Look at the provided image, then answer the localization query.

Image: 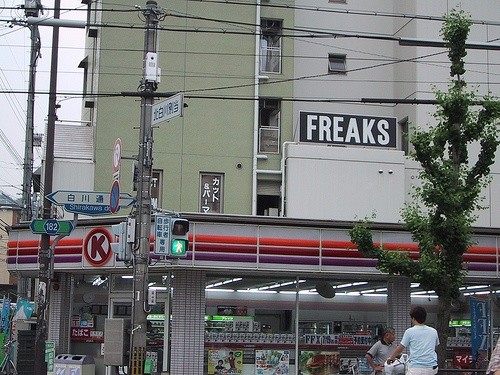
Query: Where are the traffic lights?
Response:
[169,218,190,258]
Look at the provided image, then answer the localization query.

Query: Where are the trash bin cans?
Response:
[54,354,95,375]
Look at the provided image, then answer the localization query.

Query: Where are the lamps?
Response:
[410,282,500,299]
[206,278,387,295]
[92,276,108,289]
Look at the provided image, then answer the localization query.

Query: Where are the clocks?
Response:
[82,293,95,303]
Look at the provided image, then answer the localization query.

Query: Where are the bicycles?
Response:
[0,340,19,375]
[386,356,411,375]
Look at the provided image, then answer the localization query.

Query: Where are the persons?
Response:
[388,306,441,375]
[365,327,402,375]
[225,352,237,368]
[485,334,500,375]
[215,360,225,372]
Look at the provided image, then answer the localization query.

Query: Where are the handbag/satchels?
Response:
[384,354,409,375]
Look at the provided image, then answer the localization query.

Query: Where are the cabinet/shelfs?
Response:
[146,315,225,339]
[447,327,472,370]
